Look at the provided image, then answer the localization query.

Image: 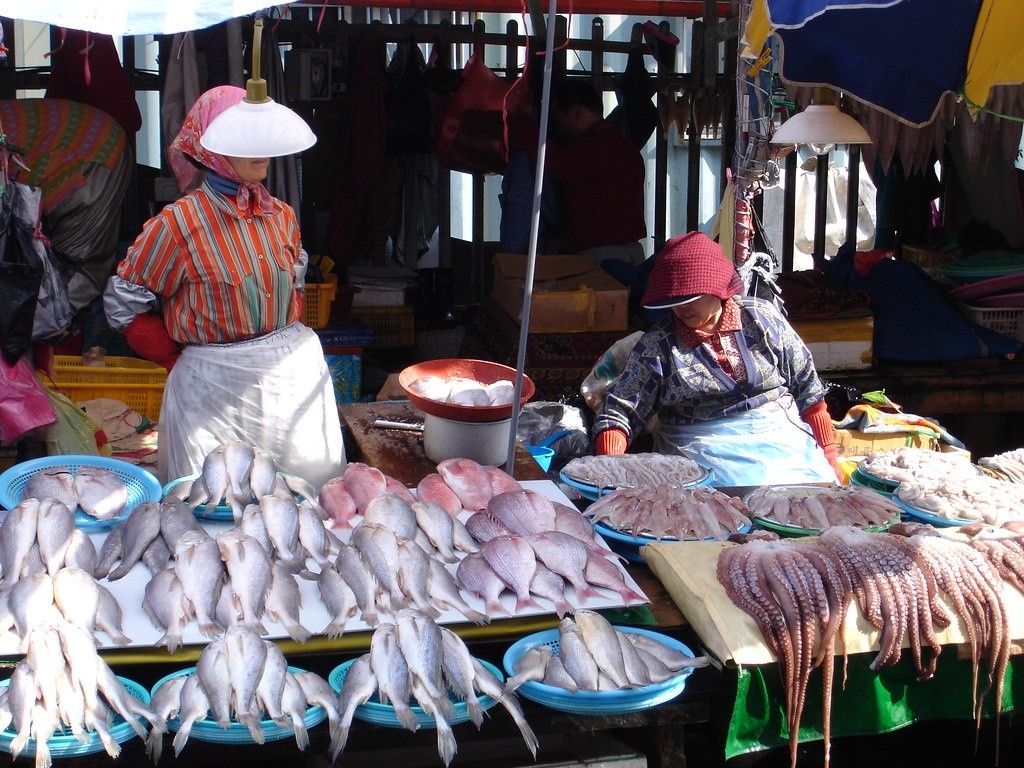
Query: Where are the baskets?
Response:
[303,275,339,330]
[957,303,1024,338]
[40,371,169,421]
[47,355,167,372]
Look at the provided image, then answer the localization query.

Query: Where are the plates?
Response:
[559,461,715,502]
[740,484,900,539]
[163,472,313,520]
[584,509,752,563]
[850,460,901,499]
[891,485,977,527]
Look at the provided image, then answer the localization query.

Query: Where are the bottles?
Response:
[769,73,789,130]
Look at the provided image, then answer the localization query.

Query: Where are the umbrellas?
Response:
[739,0,1024,130]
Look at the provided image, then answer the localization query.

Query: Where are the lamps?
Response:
[769,87,873,157]
[200,17,317,160]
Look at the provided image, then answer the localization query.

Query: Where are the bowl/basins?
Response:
[414,406,523,467]
[398,358,535,422]
[524,445,554,474]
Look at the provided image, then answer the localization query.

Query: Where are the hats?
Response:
[555,83,606,116]
[642,294,703,310]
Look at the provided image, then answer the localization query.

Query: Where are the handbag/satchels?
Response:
[33,238,73,343]
[0,183,45,367]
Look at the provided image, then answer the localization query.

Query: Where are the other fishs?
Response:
[750,484,905,526]
[1,372,750,766]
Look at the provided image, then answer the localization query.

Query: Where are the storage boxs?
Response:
[351,284,404,306]
[494,254,629,331]
[300,274,336,329]
[787,315,875,371]
[35,354,168,424]
[360,307,416,348]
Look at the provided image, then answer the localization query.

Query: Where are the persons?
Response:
[0,98,127,356]
[592,231,845,489]
[102,84,346,495]
[509,84,647,268]
